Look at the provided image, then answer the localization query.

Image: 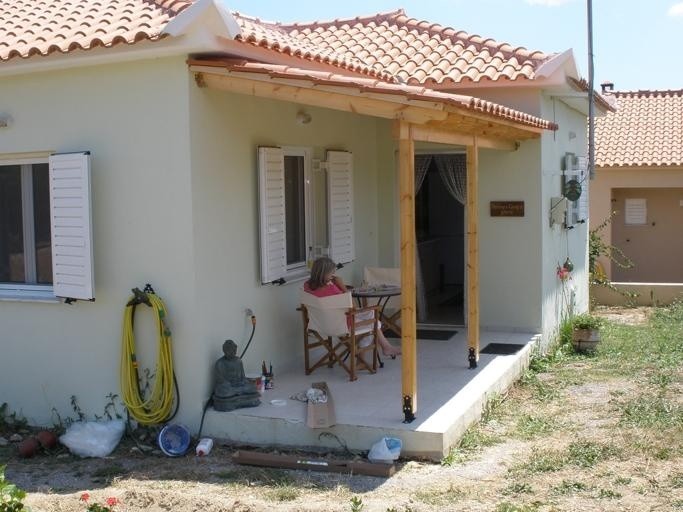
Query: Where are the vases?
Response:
[16,429,56,458]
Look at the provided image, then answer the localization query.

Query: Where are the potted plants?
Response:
[571,311,606,352]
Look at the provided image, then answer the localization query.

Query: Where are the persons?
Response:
[214,339,258,398]
[303,257,401,370]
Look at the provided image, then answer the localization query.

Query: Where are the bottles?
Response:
[196,439,212,458]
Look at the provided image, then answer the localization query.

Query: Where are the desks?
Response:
[339,285,401,369]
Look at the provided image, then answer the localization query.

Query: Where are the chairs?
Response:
[296,303,384,382]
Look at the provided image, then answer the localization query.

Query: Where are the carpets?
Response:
[381,328,458,341]
[479,343,525,356]
